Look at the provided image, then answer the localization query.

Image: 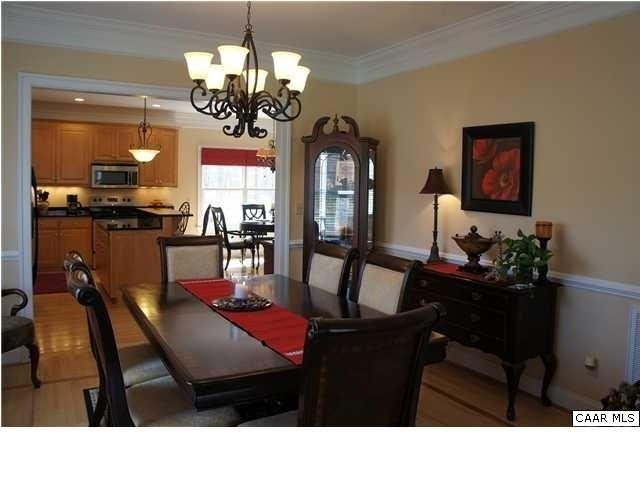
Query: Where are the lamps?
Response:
[128,97,161,164]
[256,120,275,173]
[183,1,311,138]
[533,221,552,285]
[419,168,453,263]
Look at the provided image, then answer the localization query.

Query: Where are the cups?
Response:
[66,194,84,214]
[231,274,249,300]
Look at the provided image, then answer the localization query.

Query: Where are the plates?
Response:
[212,295,273,313]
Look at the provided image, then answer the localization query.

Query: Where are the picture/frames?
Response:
[461,122,534,216]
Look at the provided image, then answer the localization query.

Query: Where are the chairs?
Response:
[2,202,445,426]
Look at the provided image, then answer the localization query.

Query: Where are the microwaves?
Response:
[91,163,139,188]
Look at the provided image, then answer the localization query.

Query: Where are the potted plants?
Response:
[502,229,552,284]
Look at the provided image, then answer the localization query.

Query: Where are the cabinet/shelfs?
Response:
[139,126,179,187]
[421,262,561,420]
[32,121,93,186]
[93,123,139,162]
[301,113,379,280]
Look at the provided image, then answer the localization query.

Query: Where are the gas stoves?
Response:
[88,207,154,219]
